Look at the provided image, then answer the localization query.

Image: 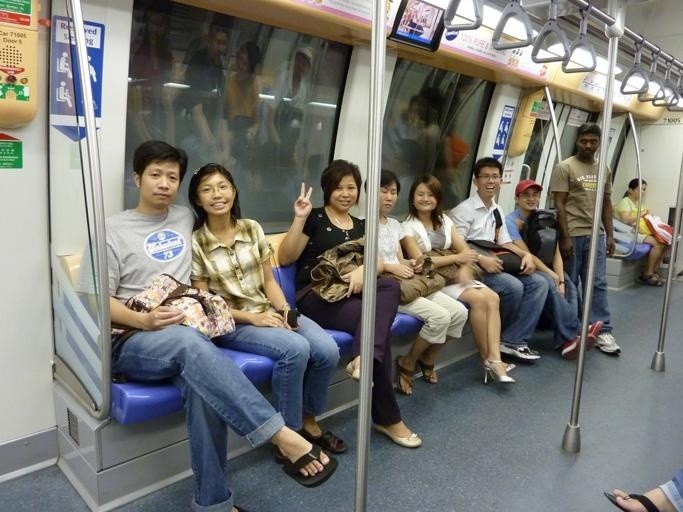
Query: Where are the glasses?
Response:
[198,184,232,195]
[477,174,501,180]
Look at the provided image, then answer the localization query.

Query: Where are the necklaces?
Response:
[331,210,353,240]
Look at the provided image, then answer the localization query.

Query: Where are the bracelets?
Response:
[559,281,566,284]
[477,255,481,261]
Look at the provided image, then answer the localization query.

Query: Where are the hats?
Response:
[513,179,544,196]
[577,120,602,137]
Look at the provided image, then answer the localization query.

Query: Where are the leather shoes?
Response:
[344,357,375,388]
[370,419,423,450]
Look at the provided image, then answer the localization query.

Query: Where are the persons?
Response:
[188,161,348,464]
[364,170,469,395]
[277,159,423,447]
[551,125,622,354]
[403,174,516,385]
[506,179,604,359]
[403,86,471,203]
[76,140,339,512]
[613,179,666,286]
[448,158,550,360]
[133,17,325,199]
[604,470,683,511]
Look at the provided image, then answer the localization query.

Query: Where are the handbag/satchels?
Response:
[464,236,526,276]
[307,234,366,306]
[421,247,483,287]
[643,213,681,247]
[377,253,447,306]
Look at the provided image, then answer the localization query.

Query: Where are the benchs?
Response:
[274,265,300,309]
[610,211,651,258]
[326,330,355,358]
[390,312,420,337]
[110,358,183,428]
[221,349,277,385]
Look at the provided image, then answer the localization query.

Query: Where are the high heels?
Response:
[481,360,516,386]
[503,362,516,374]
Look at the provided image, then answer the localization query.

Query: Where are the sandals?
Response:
[271,446,291,465]
[652,271,666,283]
[634,275,663,287]
[297,427,349,456]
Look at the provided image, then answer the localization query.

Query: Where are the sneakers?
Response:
[498,336,541,361]
[560,334,596,360]
[586,320,604,338]
[592,332,622,355]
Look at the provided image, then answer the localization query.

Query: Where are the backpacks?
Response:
[123,272,236,346]
[521,208,561,267]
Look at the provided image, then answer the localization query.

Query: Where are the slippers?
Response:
[267,431,341,489]
[394,354,413,398]
[603,490,659,512]
[417,359,439,384]
